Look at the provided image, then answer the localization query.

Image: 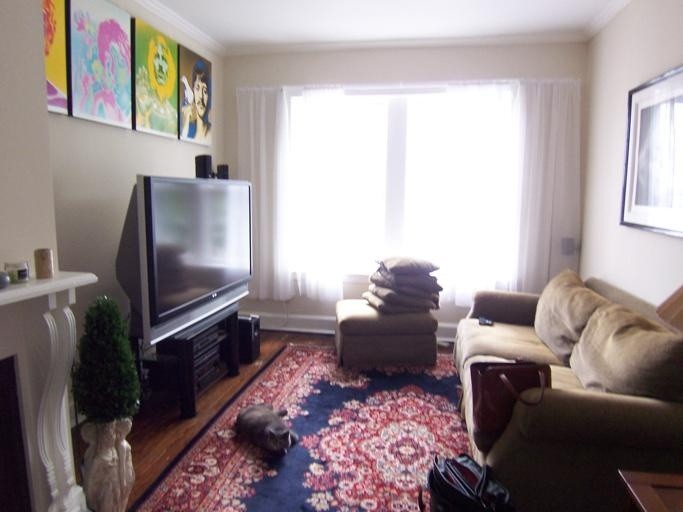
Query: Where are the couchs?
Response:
[453,278,683,512]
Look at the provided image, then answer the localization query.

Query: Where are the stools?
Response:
[335,298,439,370]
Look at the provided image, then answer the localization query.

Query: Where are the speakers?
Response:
[217,164,229,179]
[195,155,214,178]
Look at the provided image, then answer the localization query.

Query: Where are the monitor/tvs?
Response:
[137,174,253,351]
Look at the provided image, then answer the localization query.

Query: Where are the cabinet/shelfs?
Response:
[173,303,240,418]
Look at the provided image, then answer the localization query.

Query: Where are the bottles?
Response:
[4,260,30,284]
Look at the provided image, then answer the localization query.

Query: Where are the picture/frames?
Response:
[71,0,134,130]
[42,1,71,116]
[135,17,180,138]
[180,44,212,148]
[619,65,682,237]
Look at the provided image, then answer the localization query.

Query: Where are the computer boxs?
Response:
[237,314,261,363]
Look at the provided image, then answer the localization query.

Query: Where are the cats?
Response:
[236,404,299,455]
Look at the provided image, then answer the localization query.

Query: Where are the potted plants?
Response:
[71,295,141,512]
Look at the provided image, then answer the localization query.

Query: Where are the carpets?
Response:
[124,342,474,512]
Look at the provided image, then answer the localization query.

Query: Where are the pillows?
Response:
[370,271,440,300]
[377,265,443,292]
[534,270,609,364]
[375,256,440,274]
[568,306,683,399]
[369,284,437,310]
[362,292,430,314]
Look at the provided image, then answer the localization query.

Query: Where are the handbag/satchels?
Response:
[470,358,552,456]
[427,452,510,512]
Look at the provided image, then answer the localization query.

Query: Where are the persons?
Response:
[140,35,178,132]
[180,59,212,140]
